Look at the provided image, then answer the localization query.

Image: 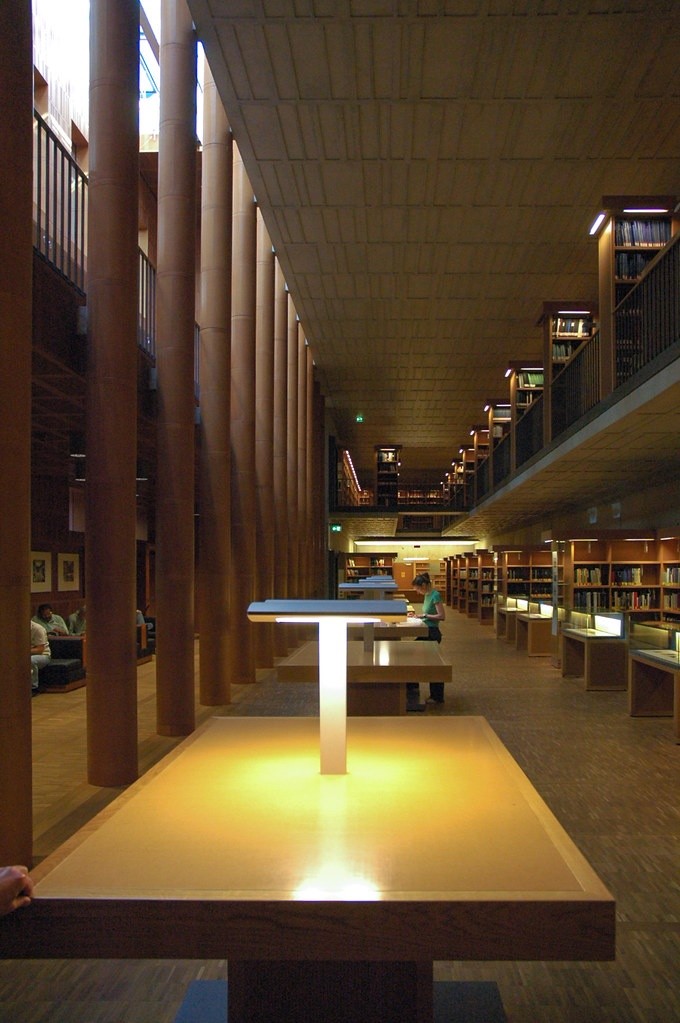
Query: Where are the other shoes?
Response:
[31,689,38,696]
[425,697,437,704]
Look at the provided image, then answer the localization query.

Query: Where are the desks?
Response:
[516,613,560,657]
[562,629,628,692]
[276,605,453,715]
[0,715,615,1023]
[497,607,527,644]
[628,649,680,746]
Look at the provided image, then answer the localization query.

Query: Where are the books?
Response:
[345,558,680,624]
[337,217,673,510]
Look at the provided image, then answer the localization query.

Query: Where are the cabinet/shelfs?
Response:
[446,526,680,630]
[342,553,398,582]
[373,445,399,505]
[413,561,446,590]
[443,195,679,505]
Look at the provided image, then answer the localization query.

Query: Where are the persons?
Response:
[407,572,446,704]
[30,605,151,697]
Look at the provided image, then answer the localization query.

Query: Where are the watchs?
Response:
[423,614,427,618]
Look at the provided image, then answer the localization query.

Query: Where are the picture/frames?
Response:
[57,552,80,592]
[30,551,52,593]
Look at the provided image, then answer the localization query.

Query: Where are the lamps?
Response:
[340,576,398,651]
[247,600,407,774]
[75,462,86,482]
[69,432,86,457]
[136,461,150,481]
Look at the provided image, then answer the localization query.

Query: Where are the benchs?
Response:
[137,616,156,665]
[38,636,86,693]
